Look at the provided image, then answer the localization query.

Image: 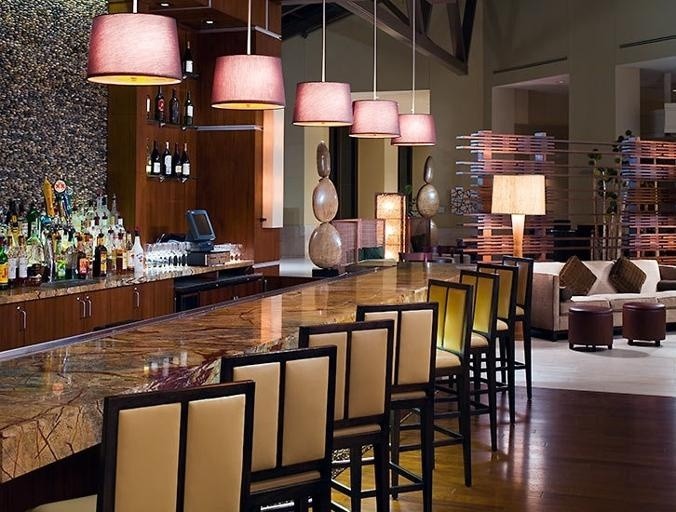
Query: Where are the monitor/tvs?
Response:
[185,210,215,239]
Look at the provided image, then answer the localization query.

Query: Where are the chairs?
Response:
[356,295,439,512]
[470,251,537,408]
[415,261,499,456]
[300,319,393,512]
[389,271,476,489]
[39,378,256,512]
[215,348,336,512]
[467,257,521,425]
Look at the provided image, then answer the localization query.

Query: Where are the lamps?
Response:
[83,1,182,87]
[256,102,284,231]
[344,0,399,141]
[211,0,288,110]
[291,0,354,125]
[374,192,409,262]
[388,0,436,147]
[483,172,549,261]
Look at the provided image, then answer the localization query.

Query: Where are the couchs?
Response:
[526,259,676,341]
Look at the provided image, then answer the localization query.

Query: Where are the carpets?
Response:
[456,332,676,401]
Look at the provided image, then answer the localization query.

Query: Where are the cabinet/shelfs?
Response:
[1,303,27,350]
[145,116,199,183]
[197,276,260,305]
[26,288,109,348]
[110,280,176,324]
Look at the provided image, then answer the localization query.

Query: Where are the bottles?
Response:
[150,140,160,175]
[214,243,240,262]
[169,86,181,124]
[154,85,164,121]
[0,194,142,289]
[145,242,186,268]
[172,143,182,178]
[184,89,193,126]
[182,40,193,75]
[161,141,172,177]
[180,143,191,178]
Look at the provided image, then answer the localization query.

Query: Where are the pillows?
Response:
[612,253,647,293]
[558,255,598,296]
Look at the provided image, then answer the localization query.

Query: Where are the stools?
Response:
[564,302,616,353]
[621,300,668,347]
